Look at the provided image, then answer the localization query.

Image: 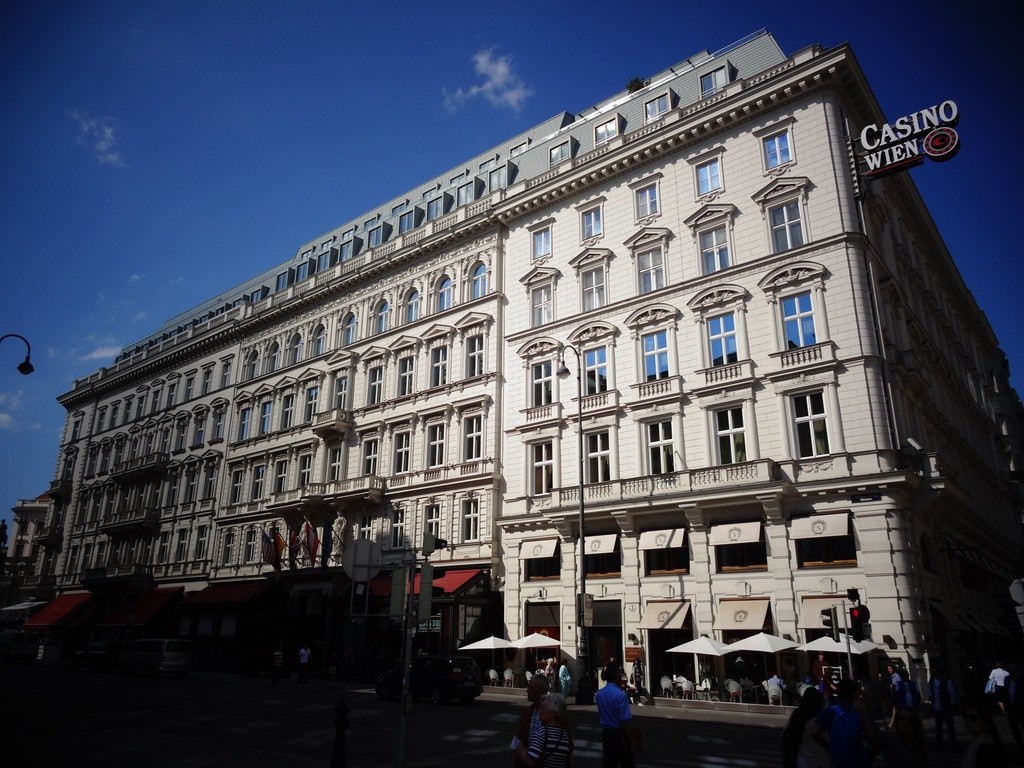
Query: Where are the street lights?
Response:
[557,344,596,706]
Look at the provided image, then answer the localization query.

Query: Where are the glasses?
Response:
[962,713,980,722]
[895,714,915,722]
[527,684,536,690]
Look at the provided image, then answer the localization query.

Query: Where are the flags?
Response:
[262,521,332,581]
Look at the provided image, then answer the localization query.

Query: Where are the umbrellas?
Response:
[664,632,880,688]
[457,631,561,670]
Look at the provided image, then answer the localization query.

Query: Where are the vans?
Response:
[117,637,194,677]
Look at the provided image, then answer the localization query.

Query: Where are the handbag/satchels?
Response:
[624,727,643,752]
[984,678,995,694]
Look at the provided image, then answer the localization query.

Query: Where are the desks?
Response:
[672,680,761,703]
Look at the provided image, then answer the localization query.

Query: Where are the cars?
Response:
[376,652,483,705]
[75,642,117,669]
[10,628,41,657]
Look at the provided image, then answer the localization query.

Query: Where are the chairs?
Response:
[695,678,712,701]
[503,670,514,688]
[489,669,498,686]
[525,671,533,682]
[681,681,694,699]
[768,685,783,705]
[660,677,673,697]
[675,676,687,698]
[727,681,743,702]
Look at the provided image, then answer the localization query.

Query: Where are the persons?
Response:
[595,662,639,768]
[297,643,313,684]
[0,519,8,548]
[761,651,1024,768]
[509,673,574,768]
[326,505,348,568]
[297,511,317,569]
[544,658,754,703]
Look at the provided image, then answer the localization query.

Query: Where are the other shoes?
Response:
[931,742,943,749]
[949,739,956,746]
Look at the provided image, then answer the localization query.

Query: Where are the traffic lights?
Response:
[423,530,447,557]
[820,606,839,642]
[420,562,448,626]
[848,606,870,643]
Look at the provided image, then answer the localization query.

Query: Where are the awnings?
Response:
[95,587,183,631]
[797,597,859,629]
[711,600,772,629]
[636,602,690,629]
[790,513,854,539]
[638,528,688,549]
[0,601,50,611]
[518,539,560,559]
[178,582,277,608]
[575,535,620,553]
[709,522,765,546]
[22,593,96,632]
[369,569,485,608]
